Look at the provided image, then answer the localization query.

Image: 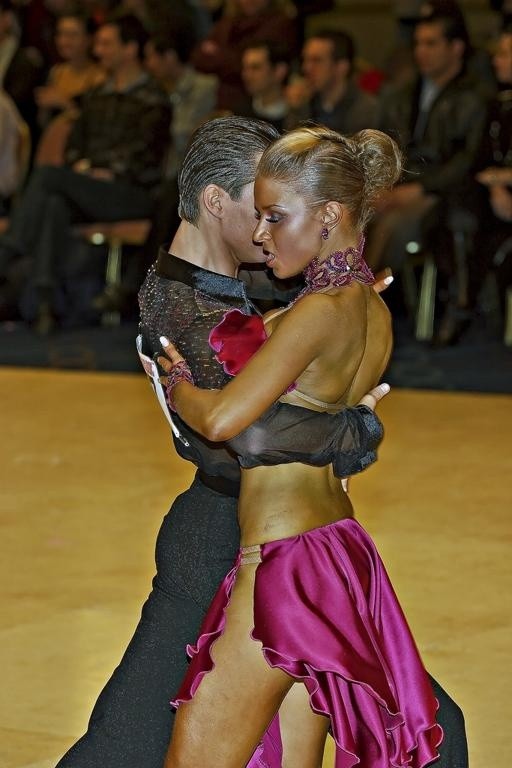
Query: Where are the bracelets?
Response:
[161,360,197,414]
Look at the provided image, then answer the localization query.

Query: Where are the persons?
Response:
[0,2,512,355]
[132,126,446,767]
[56,111,470,764]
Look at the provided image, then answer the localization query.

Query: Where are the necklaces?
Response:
[283,237,376,306]
